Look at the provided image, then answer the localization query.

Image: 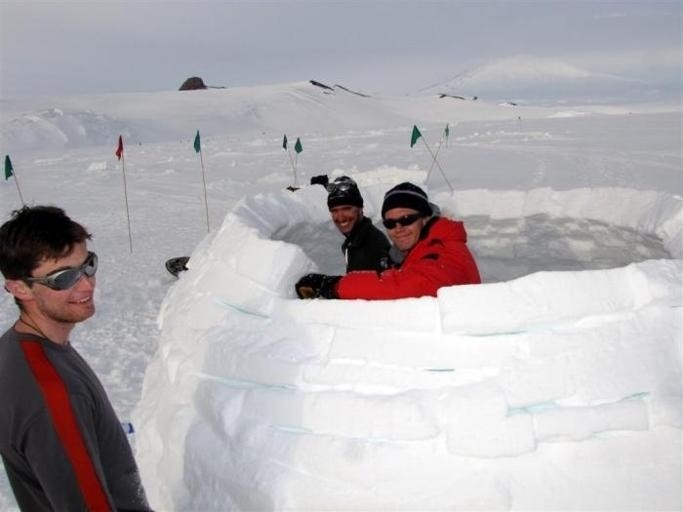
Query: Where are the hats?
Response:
[382,183,432,219]
[328,176,363,208]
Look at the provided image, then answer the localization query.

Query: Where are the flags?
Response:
[4,155,13,182]
[410,125,421,148]
[193,130,201,153]
[115,135,125,161]
[282,134,288,149]
[294,137,303,154]
[444,124,450,147]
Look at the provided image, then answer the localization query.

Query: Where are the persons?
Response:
[325,175,392,272]
[293,181,482,301]
[1,204,154,511]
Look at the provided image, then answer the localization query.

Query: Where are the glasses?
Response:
[324,183,357,192]
[21,251,98,290]
[383,213,427,229]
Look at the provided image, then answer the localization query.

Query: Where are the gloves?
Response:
[295,274,343,298]
